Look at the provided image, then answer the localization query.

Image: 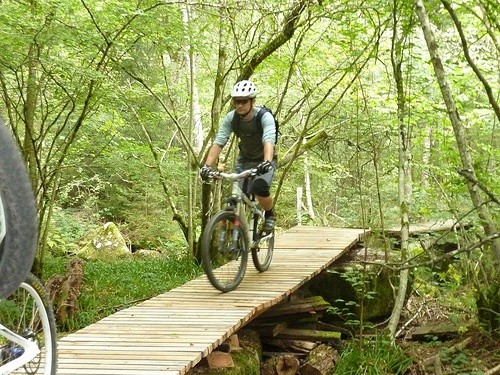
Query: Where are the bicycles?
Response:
[0,271,58,375]
[200,168,275,292]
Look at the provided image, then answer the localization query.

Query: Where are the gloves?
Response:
[257,160,273,176]
[200,164,211,181]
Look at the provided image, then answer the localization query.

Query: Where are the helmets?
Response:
[232,80,257,99]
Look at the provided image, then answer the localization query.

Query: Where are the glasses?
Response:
[233,99,251,105]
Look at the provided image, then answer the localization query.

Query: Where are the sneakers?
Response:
[264,213,276,231]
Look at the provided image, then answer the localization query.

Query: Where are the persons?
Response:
[200,80,285,233]
